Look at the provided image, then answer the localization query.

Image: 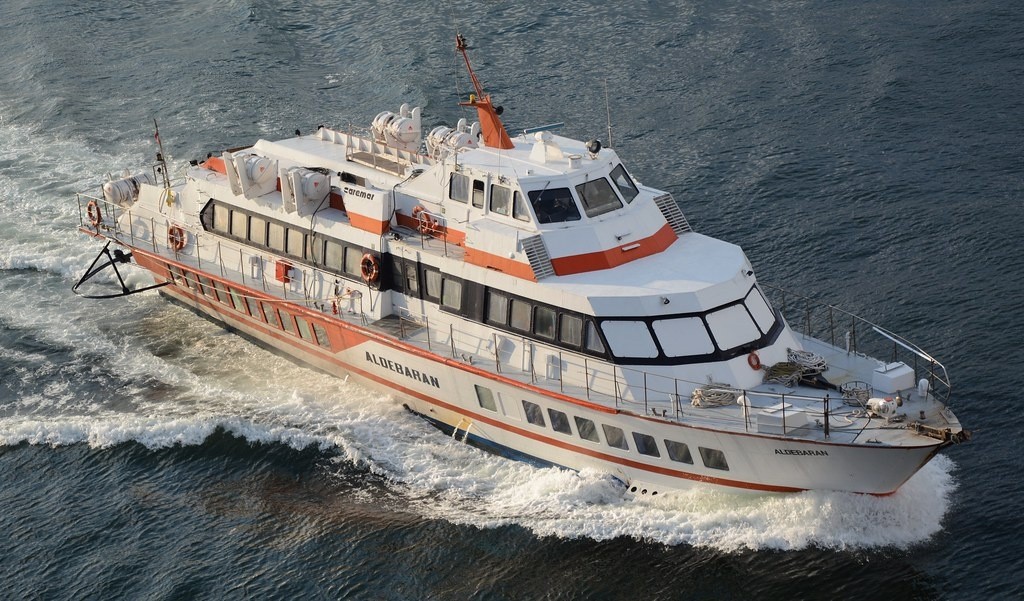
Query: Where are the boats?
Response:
[72,27,964,500]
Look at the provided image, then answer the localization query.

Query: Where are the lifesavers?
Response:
[87,201,102,228]
[169,224,183,250]
[747,354,761,370]
[411,206,432,235]
[358,253,380,282]
